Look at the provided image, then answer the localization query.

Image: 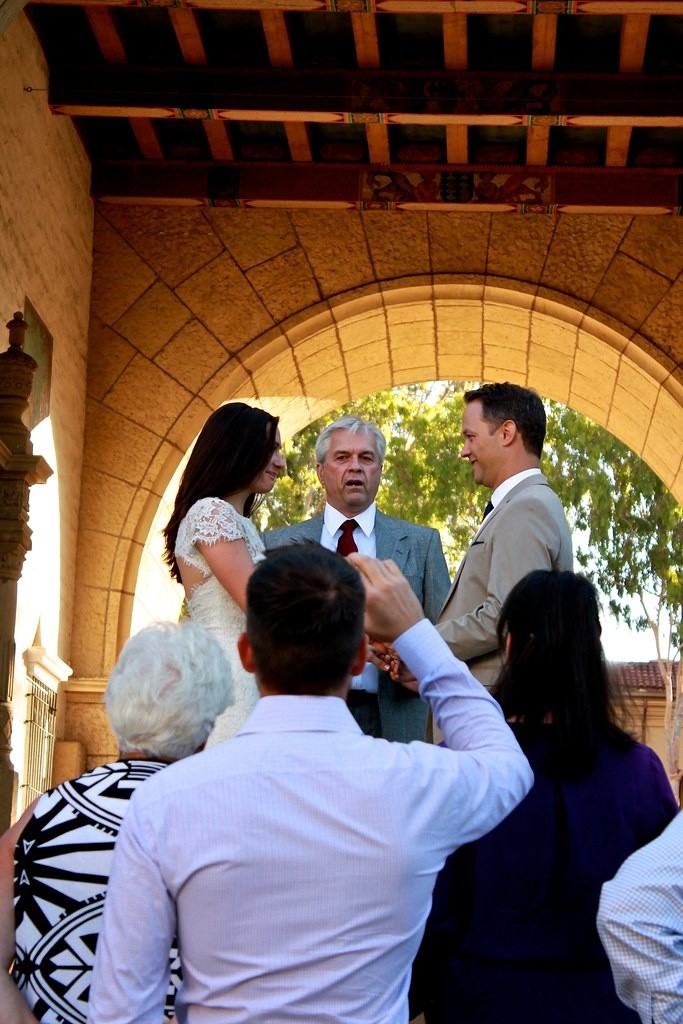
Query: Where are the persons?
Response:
[158,402,286,753]
[87,540,537,1024]
[407,568,683,1024]
[0,619,239,1024]
[254,415,452,744]
[596,808,683,1024]
[432,382,573,696]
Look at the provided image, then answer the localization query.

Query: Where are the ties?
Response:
[481,501,495,521]
[337,520,360,558]
